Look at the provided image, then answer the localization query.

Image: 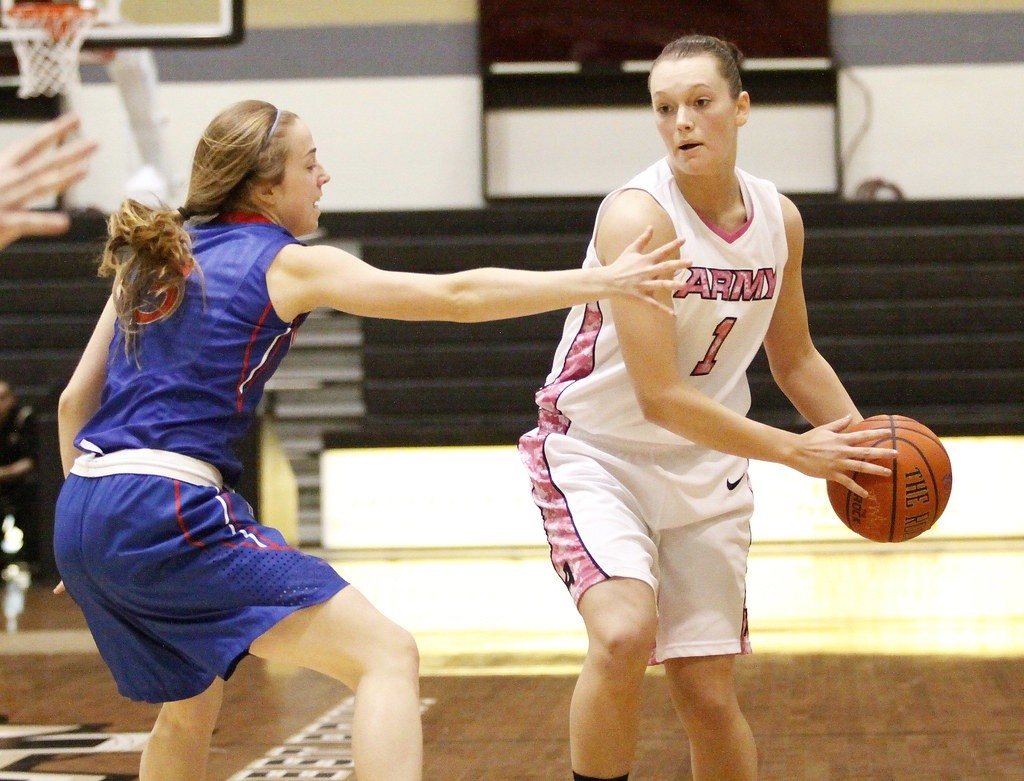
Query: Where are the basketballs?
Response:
[826,416,954,544]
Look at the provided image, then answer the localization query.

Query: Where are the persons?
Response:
[0,379,51,587]
[53,98,693,781]
[0,110,100,252]
[517,31,900,781]
